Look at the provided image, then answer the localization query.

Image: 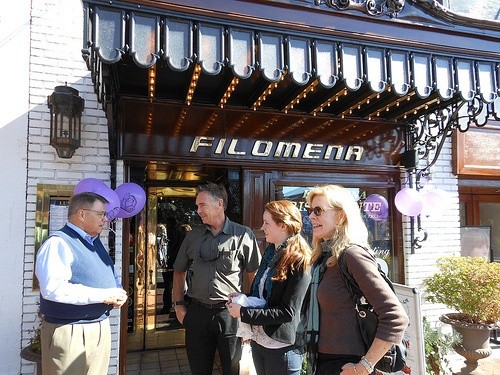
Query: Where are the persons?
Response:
[305,184,410,375]
[161,224,193,313]
[173,183,262,375]
[34,192,128,375]
[225,200,312,375]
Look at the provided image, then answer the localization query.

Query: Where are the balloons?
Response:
[394,187,423,217]
[362,193,389,221]
[75,179,147,222]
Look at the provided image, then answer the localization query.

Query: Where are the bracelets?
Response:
[172,301,188,310]
[359,356,374,374]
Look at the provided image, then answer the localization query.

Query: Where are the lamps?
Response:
[46,81,85,159]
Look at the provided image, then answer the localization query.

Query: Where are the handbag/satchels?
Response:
[337,244,411,374]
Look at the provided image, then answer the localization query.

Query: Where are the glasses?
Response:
[82,208,107,218]
[198,237,220,262]
[307,206,327,217]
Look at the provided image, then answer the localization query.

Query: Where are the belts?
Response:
[189,297,226,311]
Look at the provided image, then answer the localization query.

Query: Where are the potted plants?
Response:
[418,254,500,352]
[20,301,42,375]
